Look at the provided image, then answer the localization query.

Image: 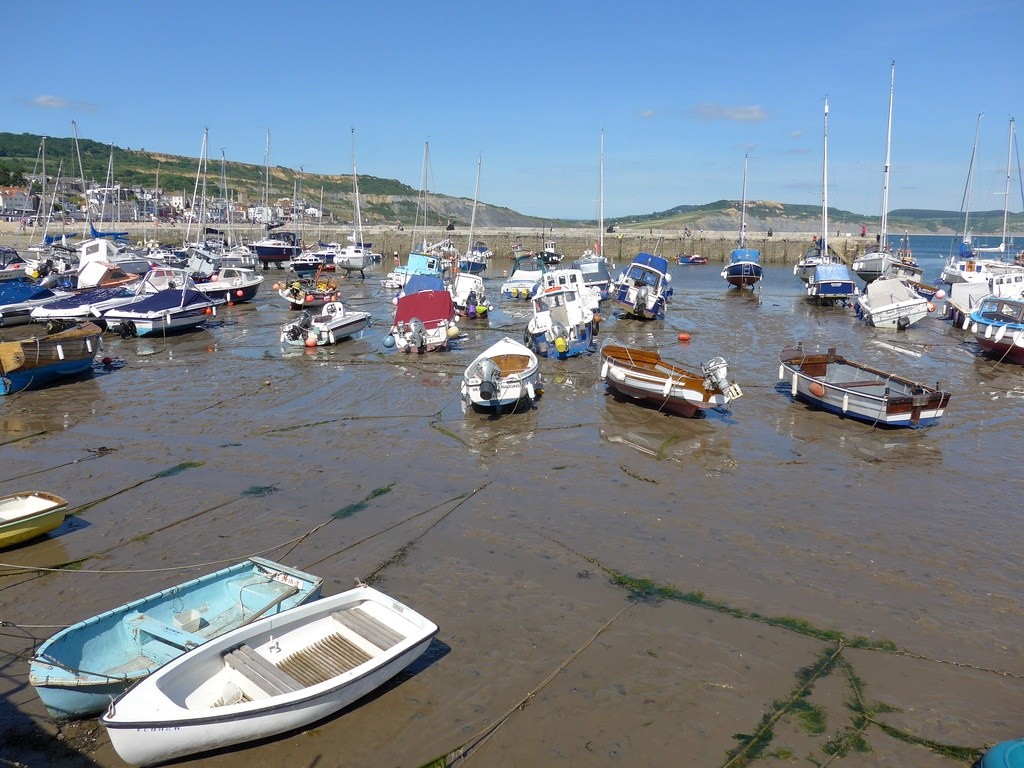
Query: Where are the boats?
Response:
[0,489,69,549]
[941,282,1024,364]
[777,341,951,430]
[853,272,937,332]
[28,554,324,723]
[499,219,616,360]
[380,252,493,354]
[460,336,546,407]
[676,254,709,265]
[599,337,744,418]
[98,576,440,767]
[805,264,858,309]
[0,238,265,397]
[606,237,674,320]
[274,274,374,347]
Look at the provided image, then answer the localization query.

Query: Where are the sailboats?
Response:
[579,128,617,269]
[12,120,188,269]
[719,154,764,286]
[405,134,493,274]
[793,98,851,281]
[939,112,1024,285]
[332,126,384,271]
[181,124,344,272]
[850,58,923,284]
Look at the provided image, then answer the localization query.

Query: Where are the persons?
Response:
[861,225,866,237]
[813,235,816,243]
[768,228,772,237]
